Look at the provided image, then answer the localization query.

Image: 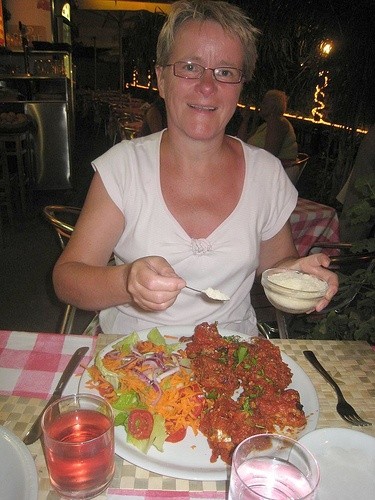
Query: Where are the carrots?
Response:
[81,340,204,437]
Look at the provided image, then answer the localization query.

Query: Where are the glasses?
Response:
[164,61,246,84]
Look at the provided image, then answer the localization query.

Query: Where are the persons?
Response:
[237,89,300,184]
[268,124,375,339]
[53,4,339,337]
[136,97,166,138]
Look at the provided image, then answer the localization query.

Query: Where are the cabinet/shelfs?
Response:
[0,50,75,190]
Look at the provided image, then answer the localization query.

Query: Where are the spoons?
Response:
[185,285,230,301]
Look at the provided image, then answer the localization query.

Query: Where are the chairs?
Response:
[294,242,375,335]
[282,152,309,183]
[60,253,289,340]
[42,206,83,250]
[0,136,19,231]
[76,89,162,147]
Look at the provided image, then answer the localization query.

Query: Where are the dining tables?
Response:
[0,330,375,500]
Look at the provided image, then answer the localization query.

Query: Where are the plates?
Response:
[77,325,319,481]
[288,428,375,500]
[0,423,38,500]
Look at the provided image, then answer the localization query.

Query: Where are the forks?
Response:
[303,351,374,426]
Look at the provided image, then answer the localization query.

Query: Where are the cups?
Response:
[40,392,117,500]
[228,433,320,500]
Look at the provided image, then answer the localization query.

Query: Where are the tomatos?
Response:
[179,384,204,400]
[165,419,186,443]
[128,410,154,440]
[186,395,208,421]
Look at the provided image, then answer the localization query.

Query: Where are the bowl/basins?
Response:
[261,268,329,314]
[0,112,33,133]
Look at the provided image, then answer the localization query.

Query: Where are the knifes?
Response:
[22,346,89,444]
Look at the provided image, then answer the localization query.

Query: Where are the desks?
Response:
[250,197,341,339]
[0,129,34,229]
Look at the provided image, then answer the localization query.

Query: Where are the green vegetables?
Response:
[95,326,274,454]
